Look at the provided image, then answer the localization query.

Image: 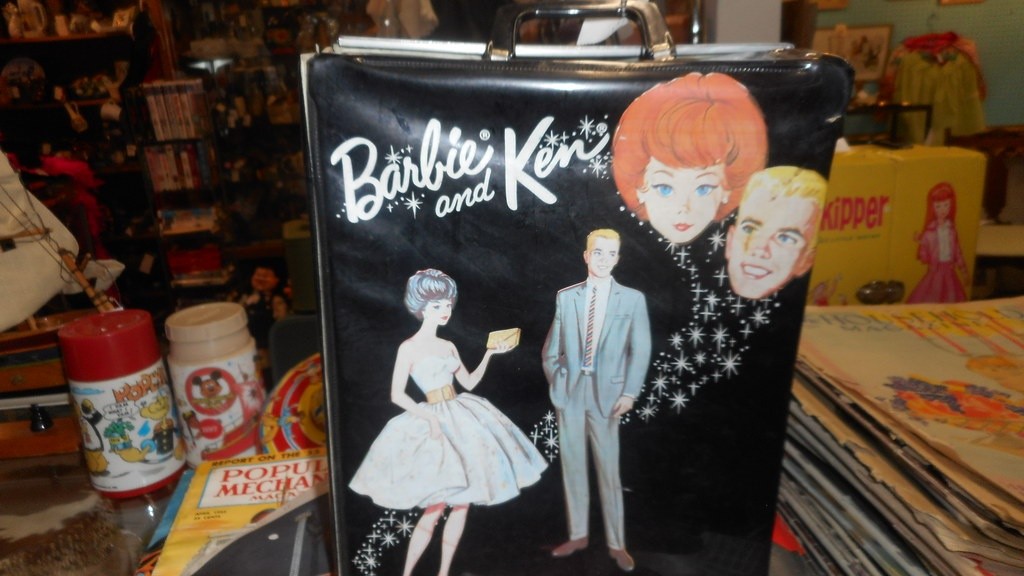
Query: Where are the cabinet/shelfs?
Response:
[0,0,336,310]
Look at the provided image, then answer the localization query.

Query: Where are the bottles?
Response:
[165,302,267,468]
[58,311,187,499]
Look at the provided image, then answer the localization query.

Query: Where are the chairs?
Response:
[267,316,323,390]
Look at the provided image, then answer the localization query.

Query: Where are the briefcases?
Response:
[300,0,854,576]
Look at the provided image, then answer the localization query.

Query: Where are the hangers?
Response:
[890,13,981,69]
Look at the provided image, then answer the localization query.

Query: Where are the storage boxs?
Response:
[803,137,987,306]
[807,23,895,85]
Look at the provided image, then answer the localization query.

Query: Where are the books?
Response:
[140,80,200,140]
[780,296,1024,576]
[144,146,198,192]
